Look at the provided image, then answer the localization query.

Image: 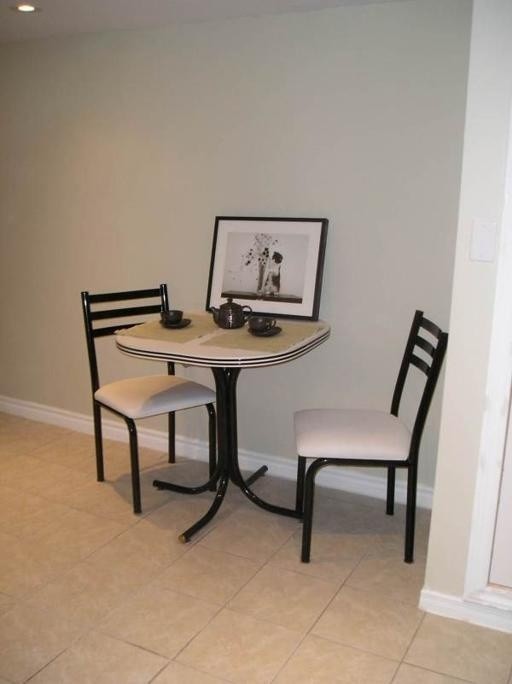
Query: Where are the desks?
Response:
[115,314,331,544]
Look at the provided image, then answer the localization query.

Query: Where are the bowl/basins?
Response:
[160,309,184,323]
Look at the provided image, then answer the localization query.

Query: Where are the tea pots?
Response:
[211,298,252,329]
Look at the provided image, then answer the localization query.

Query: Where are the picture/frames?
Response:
[206,217,329,320]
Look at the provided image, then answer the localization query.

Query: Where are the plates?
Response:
[250,326,281,336]
[159,318,191,327]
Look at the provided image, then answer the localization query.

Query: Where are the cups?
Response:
[247,316,276,329]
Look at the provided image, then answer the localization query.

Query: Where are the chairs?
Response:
[294,310,448,563]
[81,284,218,514]
